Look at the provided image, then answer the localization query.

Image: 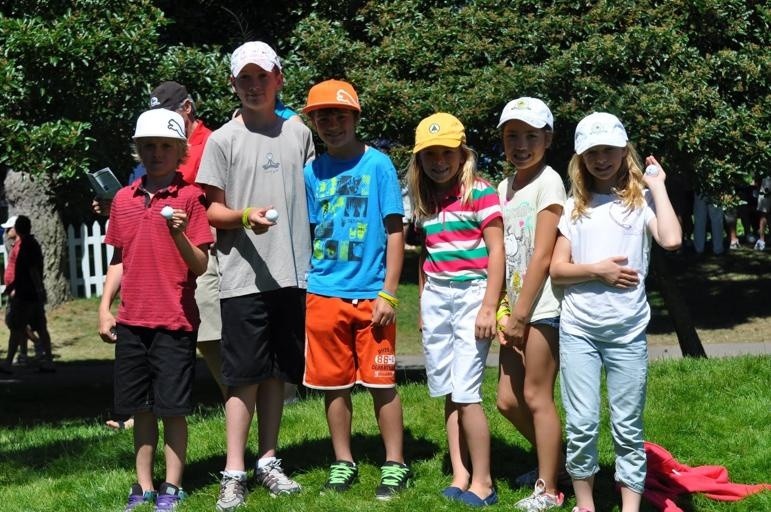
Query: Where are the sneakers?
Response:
[453,488,498,509]
[216,467,250,512]
[0,351,56,373]
[515,455,572,488]
[155,481,188,512]
[728,232,767,252]
[320,459,359,498]
[123,483,156,512]
[373,458,414,501]
[253,456,304,499]
[440,479,466,505]
[513,478,565,512]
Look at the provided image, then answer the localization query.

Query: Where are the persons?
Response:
[495,96,568,512]
[548,112,684,512]
[0,214,56,375]
[408,112,506,509]
[668,168,771,256]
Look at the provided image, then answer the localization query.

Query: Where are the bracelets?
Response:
[496,307,512,321]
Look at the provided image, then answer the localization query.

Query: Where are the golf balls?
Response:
[160,206,174,220]
[645,165,658,177]
[266,209,279,223]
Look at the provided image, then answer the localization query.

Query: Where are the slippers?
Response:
[105,416,135,432]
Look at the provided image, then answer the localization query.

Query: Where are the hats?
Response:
[573,111,628,155]
[301,79,362,118]
[412,111,467,154]
[131,107,188,142]
[150,81,188,112]
[230,40,282,79]
[0,215,19,228]
[496,96,554,136]
